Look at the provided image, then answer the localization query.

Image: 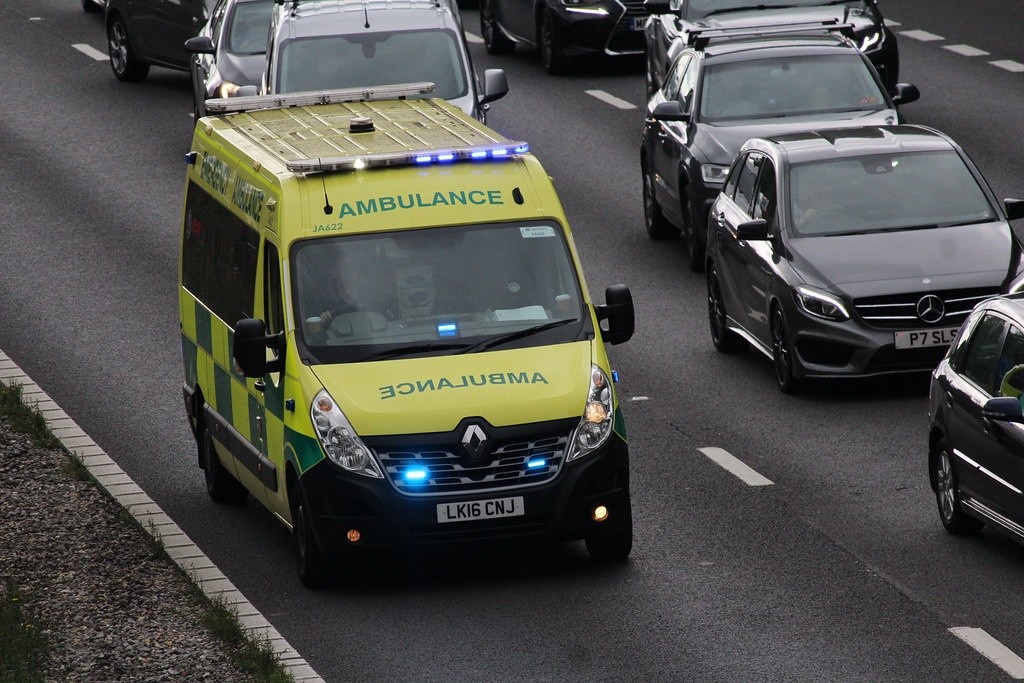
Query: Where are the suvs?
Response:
[637,23,921,275]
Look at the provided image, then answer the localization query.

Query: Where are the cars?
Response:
[642,0,900,100]
[926,293,1024,551]
[479,0,656,80]
[105,0,218,82]
[704,123,1019,399]
[183,0,278,124]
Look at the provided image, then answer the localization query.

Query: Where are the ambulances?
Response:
[179,81,634,595]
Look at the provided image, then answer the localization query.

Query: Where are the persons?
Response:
[319,256,364,327]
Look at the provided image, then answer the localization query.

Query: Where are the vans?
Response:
[236,0,511,124]
